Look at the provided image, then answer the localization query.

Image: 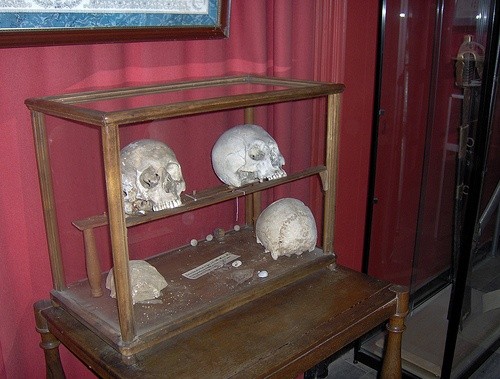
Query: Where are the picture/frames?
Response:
[0,1,231,49]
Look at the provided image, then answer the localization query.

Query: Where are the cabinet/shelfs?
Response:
[24,71,351,356]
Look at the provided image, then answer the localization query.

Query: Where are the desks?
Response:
[32,233,409,379]
